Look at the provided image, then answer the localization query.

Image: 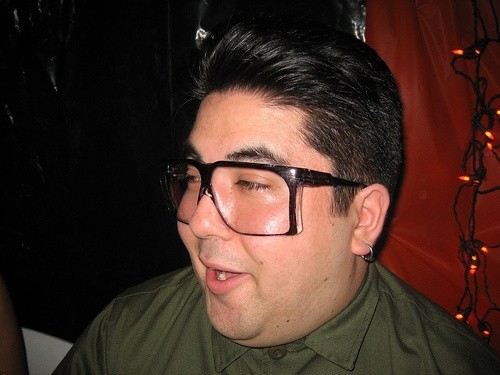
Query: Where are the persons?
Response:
[53,20,500,375]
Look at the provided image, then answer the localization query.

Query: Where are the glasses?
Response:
[158,159,368,236]
